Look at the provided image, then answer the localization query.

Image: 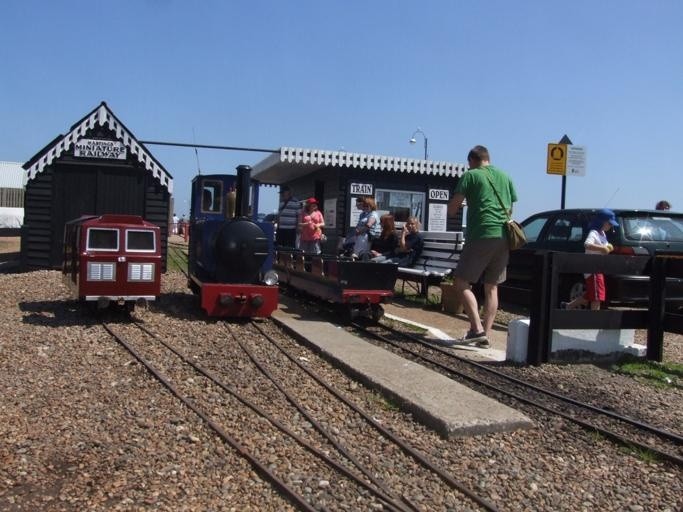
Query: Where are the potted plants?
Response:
[438,273,472,314]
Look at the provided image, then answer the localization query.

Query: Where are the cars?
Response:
[472,208,683,328]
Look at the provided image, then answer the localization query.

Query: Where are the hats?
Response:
[588,207,619,230]
[305,197,319,205]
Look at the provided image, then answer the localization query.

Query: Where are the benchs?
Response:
[379,229,464,304]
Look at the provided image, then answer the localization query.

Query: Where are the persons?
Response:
[277,187,302,247]
[656,201,670,211]
[172,214,179,233]
[343,196,422,267]
[560,209,620,311]
[297,196,325,255]
[449,146,517,349]
[180,214,187,235]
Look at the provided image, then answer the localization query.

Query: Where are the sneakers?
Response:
[559,299,570,311]
[457,328,494,348]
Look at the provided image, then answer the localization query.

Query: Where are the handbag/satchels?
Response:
[503,218,528,251]
